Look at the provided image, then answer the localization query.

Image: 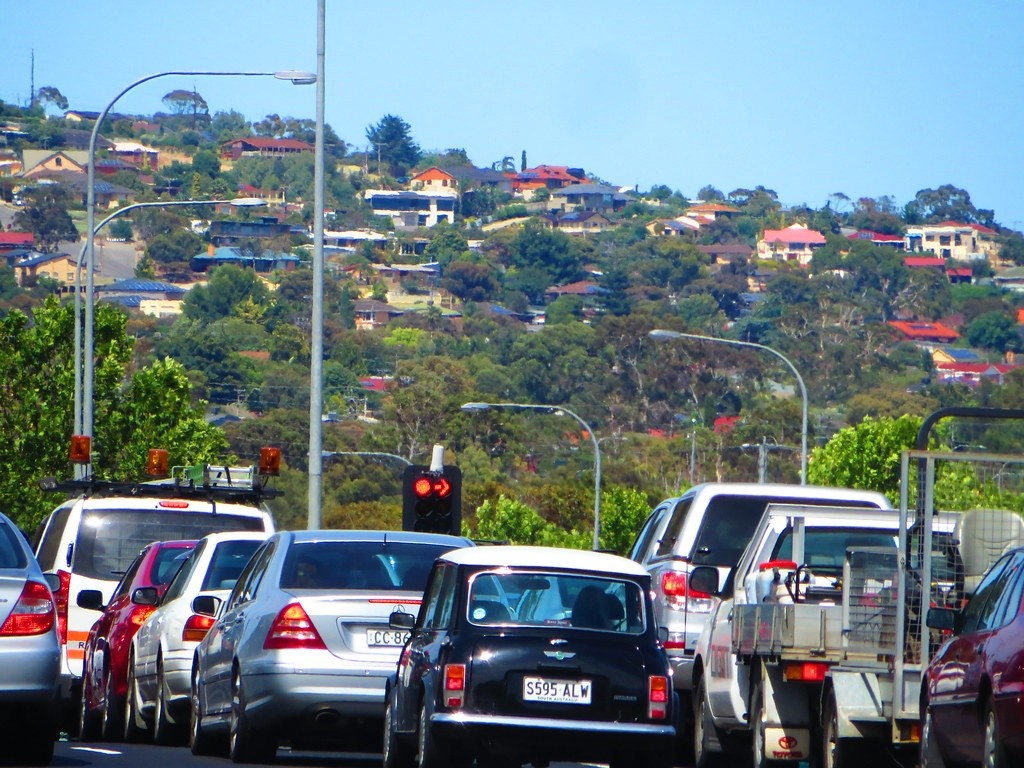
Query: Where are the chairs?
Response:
[571,592,622,629]
[474,600,512,622]
[934,508,1024,598]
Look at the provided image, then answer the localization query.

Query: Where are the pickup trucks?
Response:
[687,407,1024,768]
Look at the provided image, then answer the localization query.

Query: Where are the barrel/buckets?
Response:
[746,560,845,607]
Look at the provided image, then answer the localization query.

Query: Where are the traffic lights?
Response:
[400,463,463,535]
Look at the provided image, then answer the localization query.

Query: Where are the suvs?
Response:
[626,496,677,566]
[33,478,277,680]
[640,482,894,693]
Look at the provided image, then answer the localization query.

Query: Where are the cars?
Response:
[0,511,63,764]
[189,529,477,763]
[381,545,682,768]
[917,546,1024,768]
[76,539,200,741]
[124,531,273,742]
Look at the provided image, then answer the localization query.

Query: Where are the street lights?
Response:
[460,401,603,552]
[306,450,414,466]
[649,328,810,485]
[82,70,319,480]
[73,196,268,482]
